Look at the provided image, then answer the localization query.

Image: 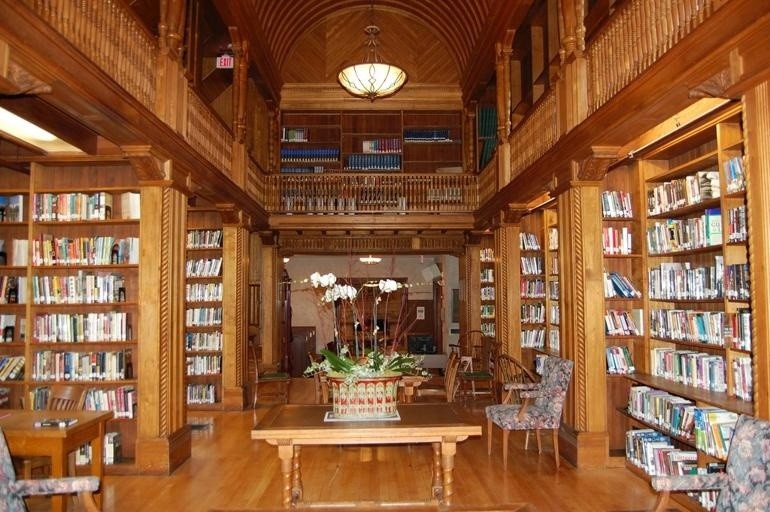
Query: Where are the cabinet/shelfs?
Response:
[1,154,248,477]
[476,109,750,512]
[281,111,471,216]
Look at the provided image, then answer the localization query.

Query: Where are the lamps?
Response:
[337,2,407,98]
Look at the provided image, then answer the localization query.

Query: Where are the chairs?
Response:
[306,351,331,403]
[417,353,461,403]
[448,330,485,364]
[650,412,770,511]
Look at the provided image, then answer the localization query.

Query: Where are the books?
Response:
[1,191,138,468]
[725,156,754,403]
[647,172,727,393]
[280,124,454,211]
[599,190,642,376]
[624,385,741,511]
[520,228,560,350]
[183,230,224,405]
[476,106,501,176]
[482,248,496,338]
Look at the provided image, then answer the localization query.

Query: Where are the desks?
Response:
[0,408,116,512]
[250,402,480,510]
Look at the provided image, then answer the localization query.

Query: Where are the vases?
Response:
[326,372,404,418]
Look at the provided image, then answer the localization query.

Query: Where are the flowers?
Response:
[276,250,436,379]
